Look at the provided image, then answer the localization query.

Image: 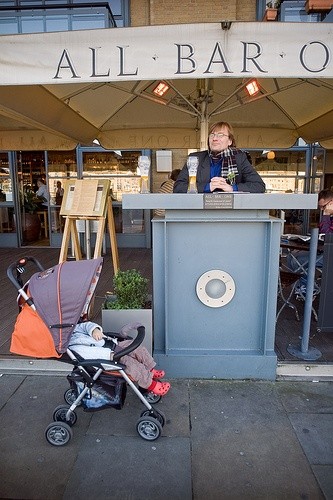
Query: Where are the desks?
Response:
[280,239,324,320]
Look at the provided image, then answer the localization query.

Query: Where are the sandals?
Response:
[151,368,165,378]
[151,381,170,395]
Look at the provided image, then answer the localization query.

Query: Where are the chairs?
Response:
[275,271,303,326]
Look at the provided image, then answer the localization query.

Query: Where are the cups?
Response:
[186,157,199,194]
[138,157,151,194]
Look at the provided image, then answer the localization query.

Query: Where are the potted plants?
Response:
[101,268,153,358]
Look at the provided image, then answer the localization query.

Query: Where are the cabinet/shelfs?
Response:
[0,157,137,204]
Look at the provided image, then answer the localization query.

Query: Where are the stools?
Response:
[38,209,63,238]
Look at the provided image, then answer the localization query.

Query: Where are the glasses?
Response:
[319,200,331,209]
[208,133,228,138]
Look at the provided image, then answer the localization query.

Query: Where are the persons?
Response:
[55,181,64,205]
[69,321,170,395]
[33,178,49,226]
[153,168,182,217]
[173,121,266,193]
[286,189,333,302]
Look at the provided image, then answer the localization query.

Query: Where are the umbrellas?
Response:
[0,22,333,152]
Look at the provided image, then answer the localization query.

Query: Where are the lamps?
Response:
[153,81,170,97]
[246,80,260,97]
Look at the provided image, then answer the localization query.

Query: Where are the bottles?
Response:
[48,158,76,163]
[87,155,138,163]
[1,159,8,164]
[22,156,45,185]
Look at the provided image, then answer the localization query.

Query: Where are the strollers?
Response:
[6,254,166,447]
